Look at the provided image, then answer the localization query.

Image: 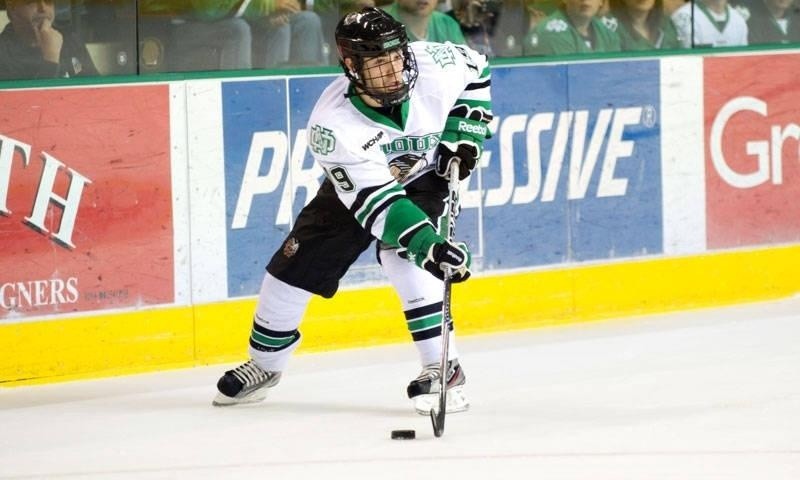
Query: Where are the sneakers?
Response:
[218,358,282,398]
[408,359,466,398]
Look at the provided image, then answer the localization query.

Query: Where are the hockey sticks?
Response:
[429,159,460,439]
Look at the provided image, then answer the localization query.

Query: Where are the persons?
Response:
[522,0,800,58]
[0,2,99,78]
[163,0,466,73]
[215,5,492,400]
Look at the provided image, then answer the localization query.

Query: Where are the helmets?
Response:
[334,6,418,105]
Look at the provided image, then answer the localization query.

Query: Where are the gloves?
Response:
[397,222,470,283]
[435,104,493,180]
